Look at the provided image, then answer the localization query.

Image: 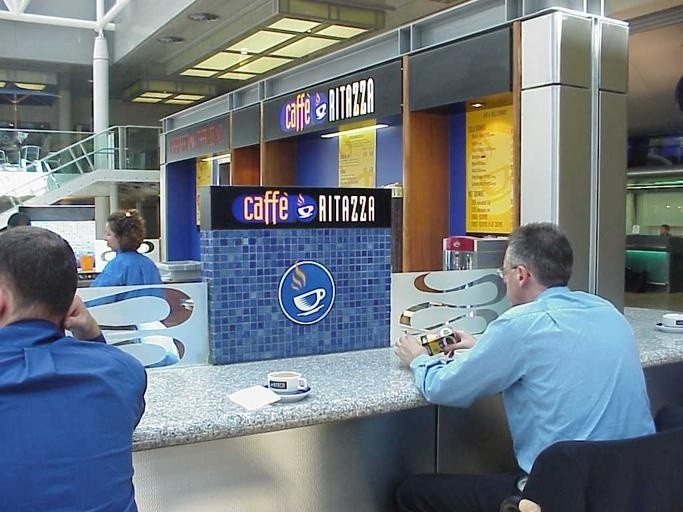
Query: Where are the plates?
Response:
[262,383,311,402]
[656,322,683,332]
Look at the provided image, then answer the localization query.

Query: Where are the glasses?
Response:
[497,264,532,280]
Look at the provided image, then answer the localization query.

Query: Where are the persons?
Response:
[90,208,165,288]
[660,225,673,236]
[1,226,150,511]
[7,212,33,233]
[40,133,53,173]
[392,222,657,512]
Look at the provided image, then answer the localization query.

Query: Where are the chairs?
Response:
[501,427,683,511]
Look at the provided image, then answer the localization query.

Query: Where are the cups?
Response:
[79,255,94,271]
[266,371,308,395]
[662,312,683,328]
